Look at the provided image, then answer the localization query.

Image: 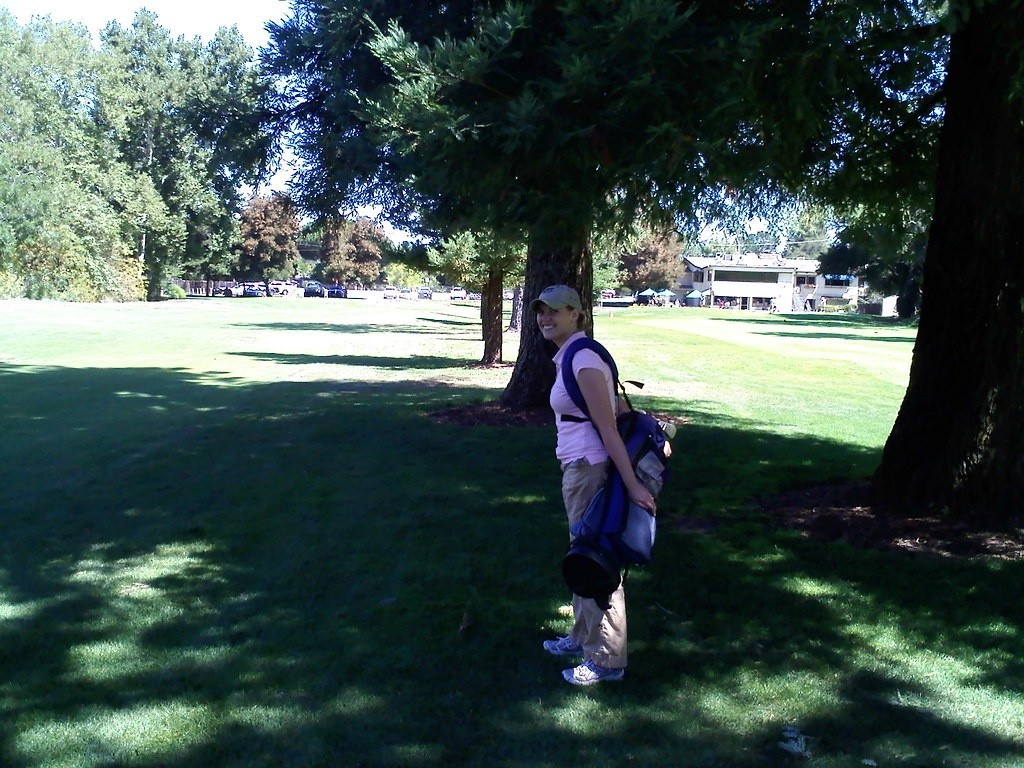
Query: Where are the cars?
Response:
[469,292,481,300]
[328,286,347,298]
[399,289,411,299]
[503,291,514,300]
[227,281,294,297]
[418,288,432,299]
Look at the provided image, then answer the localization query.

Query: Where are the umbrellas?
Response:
[657,288,676,296]
[639,288,658,296]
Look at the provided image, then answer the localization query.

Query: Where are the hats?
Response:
[529,284,583,314]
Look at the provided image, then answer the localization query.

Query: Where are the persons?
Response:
[530,285,656,686]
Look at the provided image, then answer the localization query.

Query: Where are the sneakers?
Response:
[543,635,586,657]
[561,659,625,685]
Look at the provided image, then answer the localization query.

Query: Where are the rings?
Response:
[651,497,654,500]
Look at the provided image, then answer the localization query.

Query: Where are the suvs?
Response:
[304,281,324,297]
[450,287,466,300]
[384,286,397,299]
